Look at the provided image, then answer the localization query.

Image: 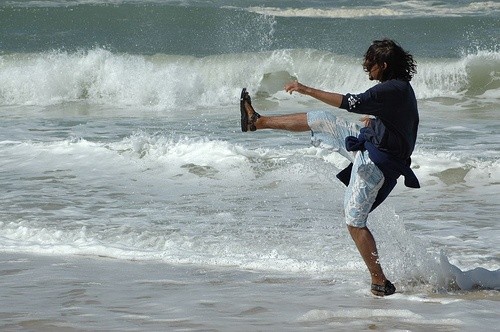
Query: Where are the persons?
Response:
[240,38,421,298]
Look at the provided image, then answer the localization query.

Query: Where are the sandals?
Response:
[239,88,261,132]
[371,280,395,297]
